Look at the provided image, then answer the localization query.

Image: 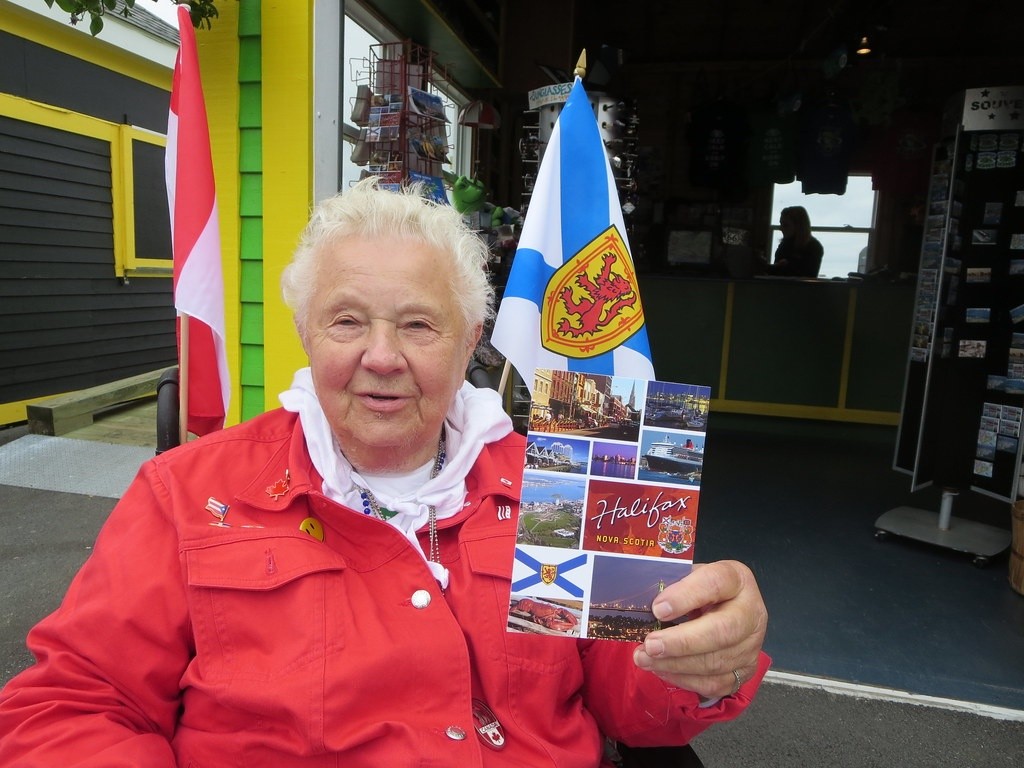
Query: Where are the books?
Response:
[910,142,1024,477]
[505,368,711,644]
[350,85,451,207]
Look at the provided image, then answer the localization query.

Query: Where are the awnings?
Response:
[581,406,603,417]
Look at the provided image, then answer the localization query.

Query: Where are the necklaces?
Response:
[356,422,507,751]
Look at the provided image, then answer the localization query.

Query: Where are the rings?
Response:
[732,670,742,694]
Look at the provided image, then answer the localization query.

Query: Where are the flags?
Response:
[165,5,233,436]
[490,74,656,399]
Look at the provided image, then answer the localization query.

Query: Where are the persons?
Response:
[775,206,823,278]
[0,176,772,768]
[543,411,606,429]
